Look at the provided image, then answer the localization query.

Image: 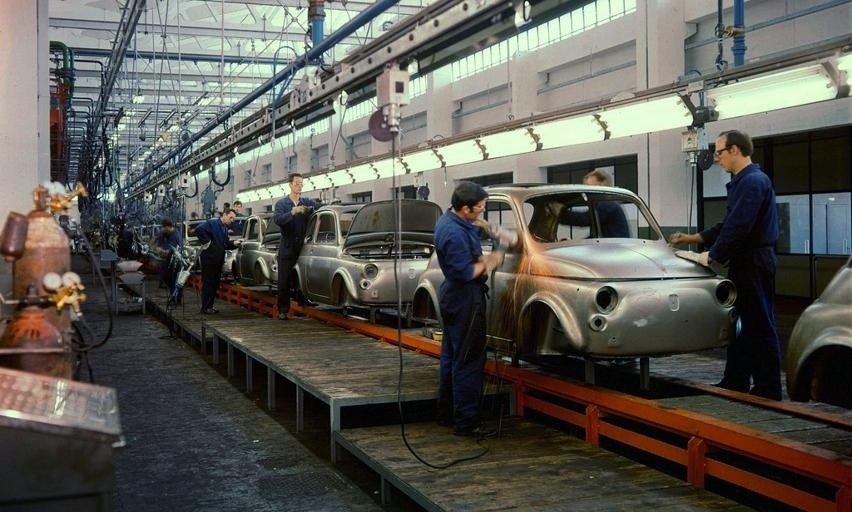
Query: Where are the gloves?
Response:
[292,206,306,214]
[489,222,518,248]
[478,249,504,276]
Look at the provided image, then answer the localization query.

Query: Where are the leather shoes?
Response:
[297,299,320,307]
[455,423,496,437]
[278,311,289,320]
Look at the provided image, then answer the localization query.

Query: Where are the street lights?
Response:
[824,197,836,254]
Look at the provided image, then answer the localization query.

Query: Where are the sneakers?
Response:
[200,306,217,315]
[211,305,220,312]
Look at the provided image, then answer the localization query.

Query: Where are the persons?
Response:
[156,222,184,305]
[672,129,783,400]
[432,182,519,438]
[558,168,630,239]
[194,209,245,314]
[227,201,247,236]
[274,172,323,319]
[220,203,230,216]
[151,219,170,289]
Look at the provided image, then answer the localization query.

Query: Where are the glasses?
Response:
[713,147,730,158]
[474,198,488,210]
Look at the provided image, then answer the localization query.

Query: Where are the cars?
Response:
[782,253,852,410]
[234,214,288,286]
[123,216,244,278]
[293,198,444,326]
[410,181,748,353]
